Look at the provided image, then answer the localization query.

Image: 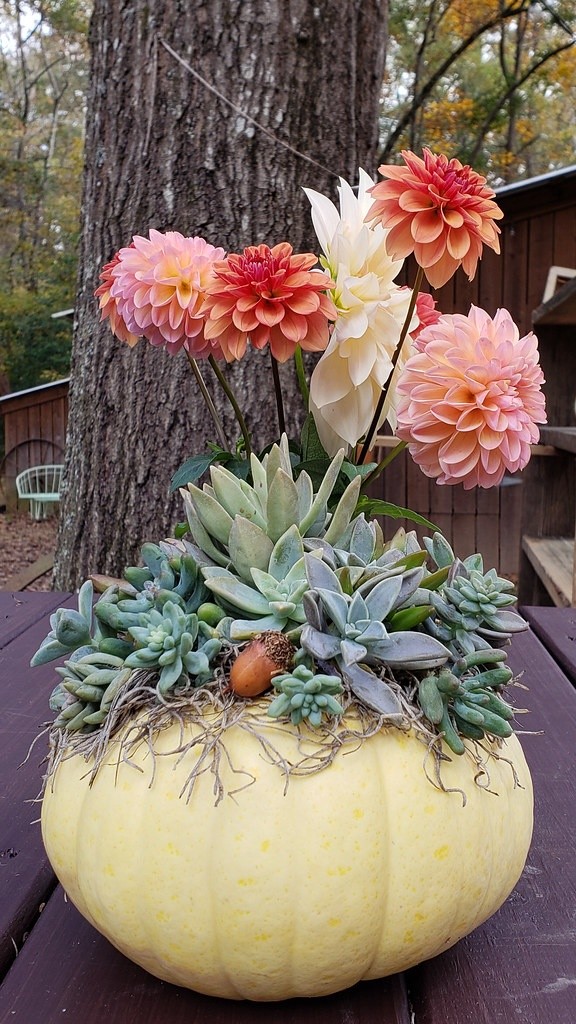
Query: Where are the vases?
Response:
[35,690,533,1003]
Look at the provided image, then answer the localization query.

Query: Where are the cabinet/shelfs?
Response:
[521,275,576,608]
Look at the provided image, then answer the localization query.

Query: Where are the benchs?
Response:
[16,464,65,523]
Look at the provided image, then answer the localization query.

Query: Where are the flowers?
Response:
[30,147,551,755]
